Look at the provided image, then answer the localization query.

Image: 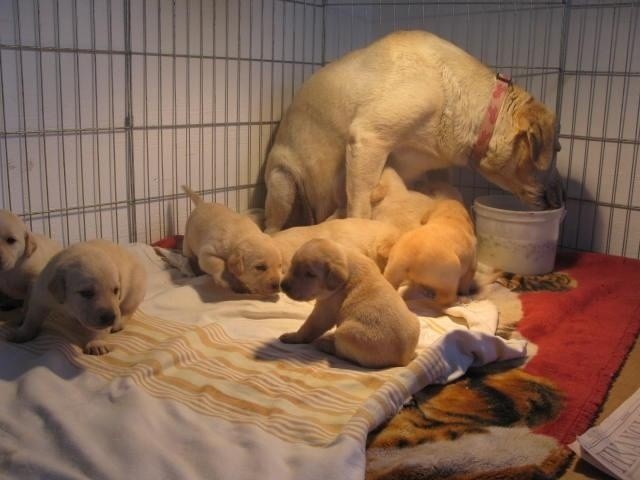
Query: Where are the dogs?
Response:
[261,26,566,241]
[0,207,58,325]
[279,239,421,369]
[266,164,481,305]
[0,239,148,357]
[176,182,285,301]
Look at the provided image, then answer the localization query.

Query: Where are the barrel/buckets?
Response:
[470,194,568,277]
